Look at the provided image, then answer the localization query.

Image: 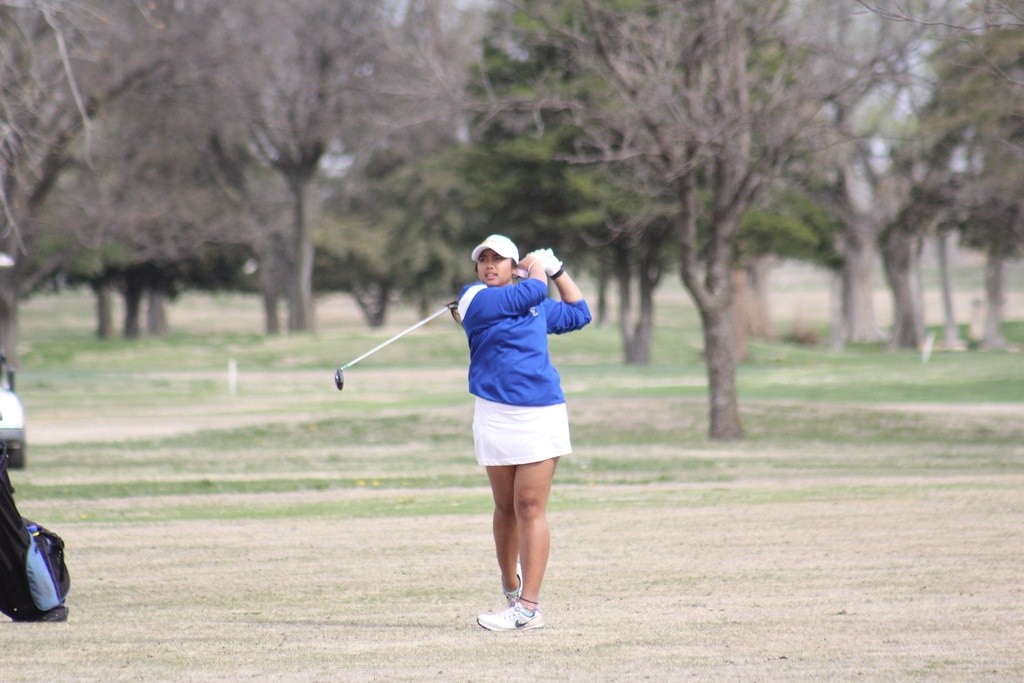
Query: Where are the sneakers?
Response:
[500,561,522,607]
[476,603,544,631]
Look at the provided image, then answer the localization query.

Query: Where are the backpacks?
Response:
[21,518,70,613]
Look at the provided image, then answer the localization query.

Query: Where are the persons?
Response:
[457,234,591,632]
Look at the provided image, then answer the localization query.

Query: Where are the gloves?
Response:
[526,248,565,279]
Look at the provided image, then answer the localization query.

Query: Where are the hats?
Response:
[471,235,519,265]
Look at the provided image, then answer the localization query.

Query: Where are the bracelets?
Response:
[528,261,537,275]
[550,268,565,279]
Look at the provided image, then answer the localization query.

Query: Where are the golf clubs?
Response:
[0,348,16,391]
[335,301,458,390]
